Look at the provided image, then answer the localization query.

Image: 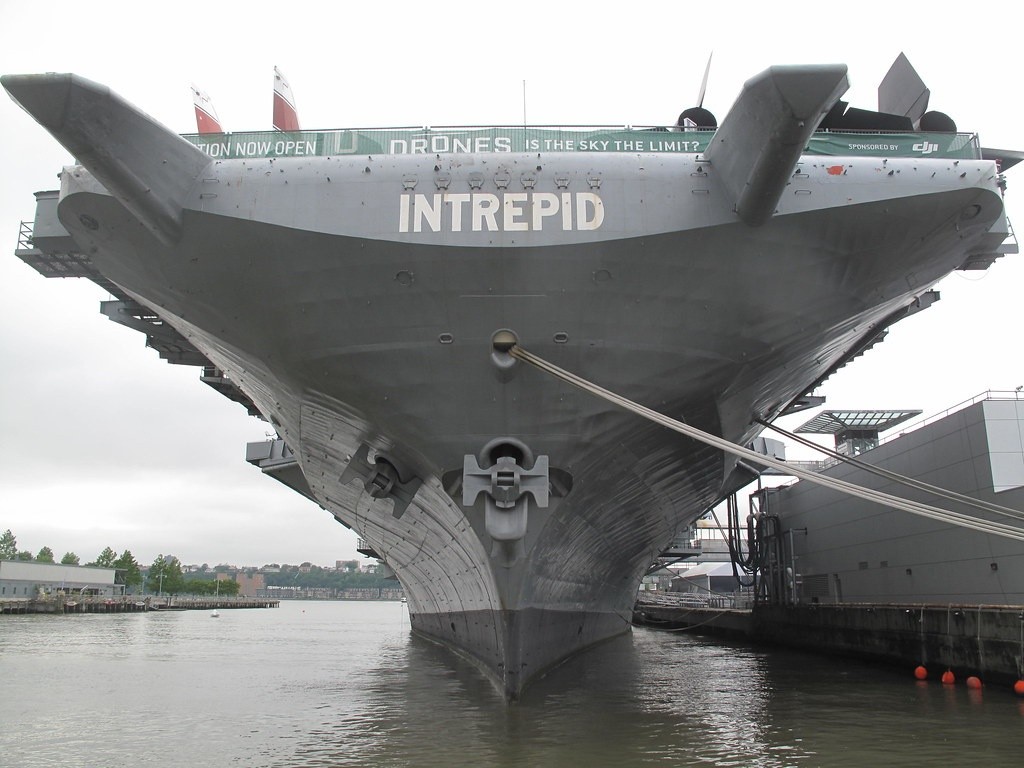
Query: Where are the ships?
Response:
[0,52,1022,712]
